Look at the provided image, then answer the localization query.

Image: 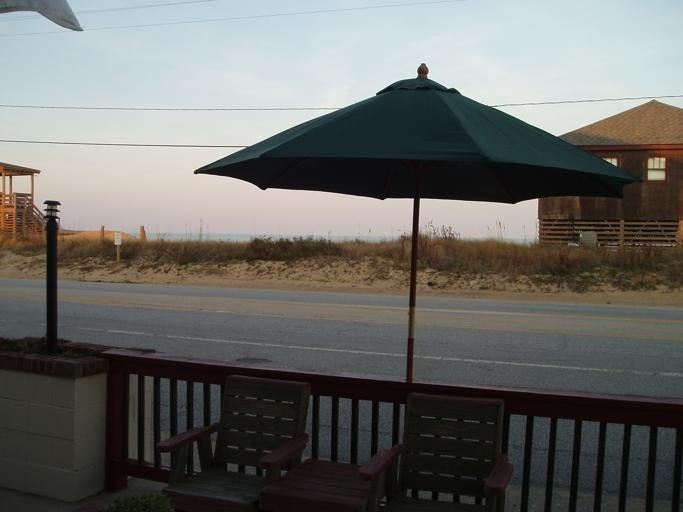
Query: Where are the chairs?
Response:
[358,391,511,510]
[156,375,312,506]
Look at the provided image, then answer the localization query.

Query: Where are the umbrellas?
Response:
[192,62,647,386]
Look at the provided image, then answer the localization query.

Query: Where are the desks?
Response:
[256,455,375,510]
